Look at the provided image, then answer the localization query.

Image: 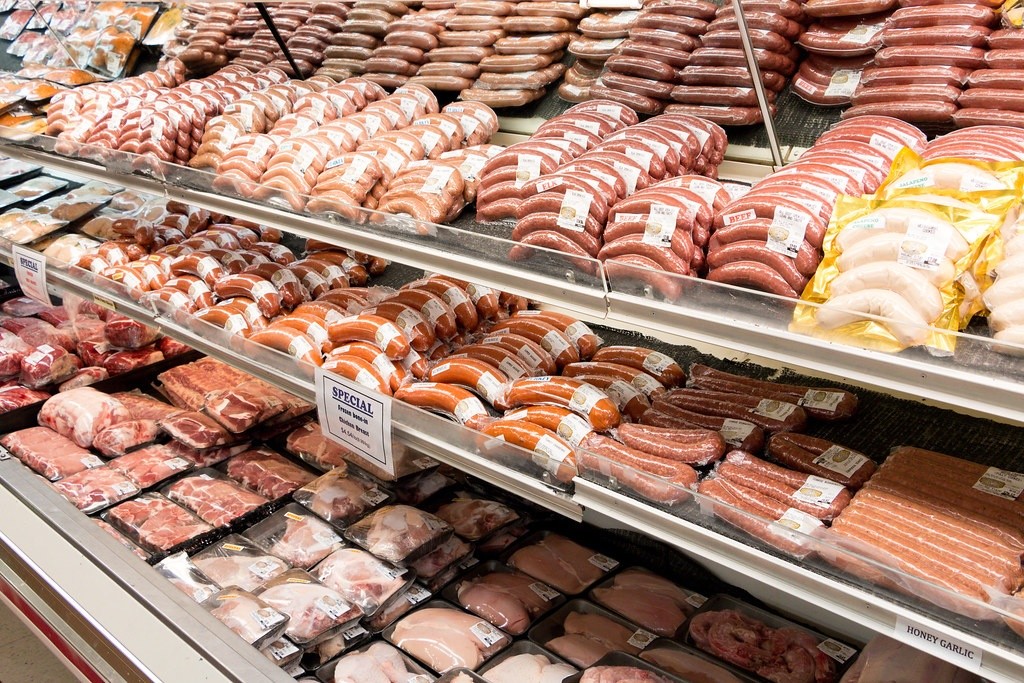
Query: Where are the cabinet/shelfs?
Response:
[0,123,1024,682]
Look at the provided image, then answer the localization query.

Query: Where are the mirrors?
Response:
[1,1,1024,170]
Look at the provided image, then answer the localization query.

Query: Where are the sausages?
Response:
[42,1,1024,640]
